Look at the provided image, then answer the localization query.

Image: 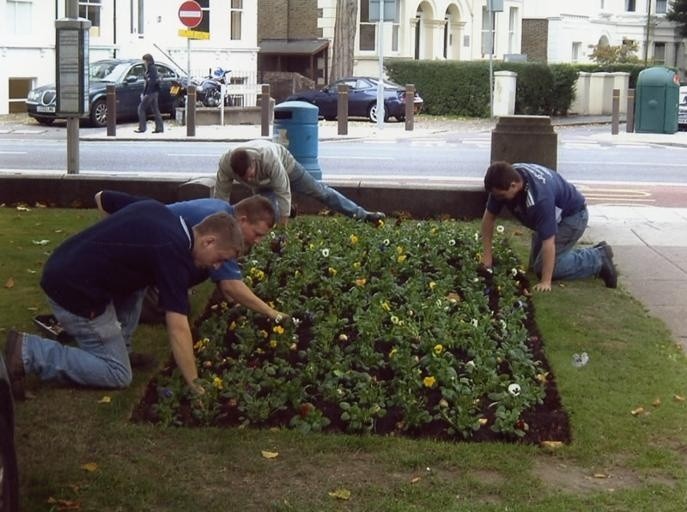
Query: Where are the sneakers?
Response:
[290,202,298,217]
[6,329,25,399]
[130,350,155,368]
[32,314,66,340]
[134,128,163,133]
[368,211,385,222]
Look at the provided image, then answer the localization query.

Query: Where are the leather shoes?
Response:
[594,240,617,289]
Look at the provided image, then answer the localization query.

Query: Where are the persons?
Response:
[211,139,386,227]
[479,163,617,293]
[113,193,291,332]
[3,199,244,405]
[133,53,165,134]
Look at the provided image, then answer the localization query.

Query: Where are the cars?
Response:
[677,85,687,125]
[22,56,185,129]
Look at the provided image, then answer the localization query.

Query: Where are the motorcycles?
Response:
[179,67,233,109]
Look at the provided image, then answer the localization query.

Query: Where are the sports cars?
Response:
[286,74,426,123]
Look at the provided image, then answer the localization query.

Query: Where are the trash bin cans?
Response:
[634,66,680,134]
[272,100,322,180]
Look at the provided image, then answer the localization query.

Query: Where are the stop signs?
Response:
[176,0,204,29]
